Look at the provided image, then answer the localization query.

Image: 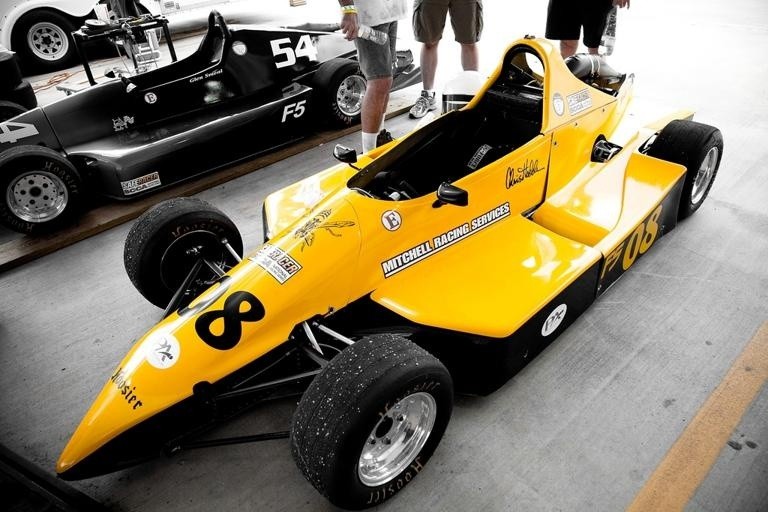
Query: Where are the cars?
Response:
[2,1,289,61]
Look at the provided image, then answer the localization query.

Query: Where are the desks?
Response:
[69,16,185,87]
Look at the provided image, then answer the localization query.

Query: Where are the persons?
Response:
[338,0,408,155]
[409,0,483,119]
[545,0,630,62]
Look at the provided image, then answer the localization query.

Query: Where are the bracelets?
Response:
[341,5,358,14]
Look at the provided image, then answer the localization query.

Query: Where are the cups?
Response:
[94,4,110,22]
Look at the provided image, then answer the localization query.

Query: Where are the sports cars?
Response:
[52,34,725,507]
[2,12,427,247]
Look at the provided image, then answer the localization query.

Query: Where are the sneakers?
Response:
[408,89,438,119]
[376,128,394,147]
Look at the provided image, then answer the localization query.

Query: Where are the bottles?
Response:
[354,24,389,46]
[596,5,619,57]
